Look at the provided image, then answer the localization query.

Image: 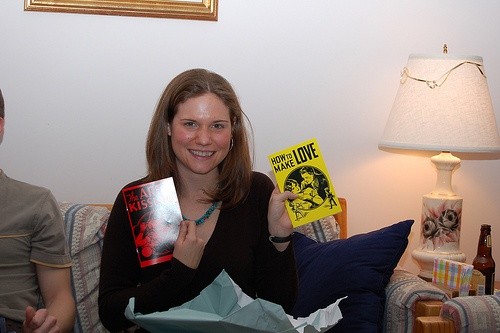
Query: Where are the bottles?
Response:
[471,224,495,298]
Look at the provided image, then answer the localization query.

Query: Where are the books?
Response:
[268,137,342,228]
[122,177,183,267]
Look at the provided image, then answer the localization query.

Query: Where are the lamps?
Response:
[378,44,500,283]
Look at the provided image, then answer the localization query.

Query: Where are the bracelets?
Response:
[269,234,292,243]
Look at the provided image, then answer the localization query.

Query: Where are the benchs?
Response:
[56,197,450,333]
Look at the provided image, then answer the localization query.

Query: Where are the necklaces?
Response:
[182,203,219,224]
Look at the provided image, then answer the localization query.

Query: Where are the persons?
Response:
[0,89,77,333]
[97,68,298,333]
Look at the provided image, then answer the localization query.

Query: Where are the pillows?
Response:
[294,218,416,333]
